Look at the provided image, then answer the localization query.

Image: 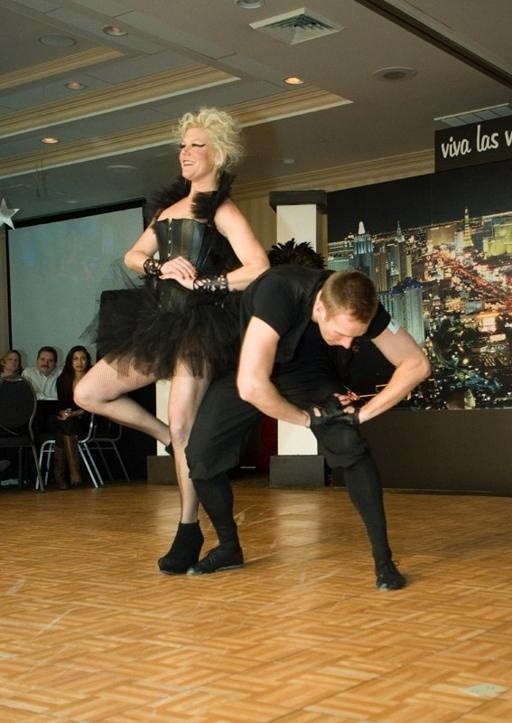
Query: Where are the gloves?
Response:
[305,393,347,428]
[331,407,360,427]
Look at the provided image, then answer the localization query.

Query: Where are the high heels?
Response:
[156,520,205,575]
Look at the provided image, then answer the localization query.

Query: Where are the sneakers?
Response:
[373,557,404,590]
[189,545,245,573]
[1,478,83,490]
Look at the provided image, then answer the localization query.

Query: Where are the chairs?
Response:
[1,375,134,492]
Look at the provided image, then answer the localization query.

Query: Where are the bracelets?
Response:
[142,258,166,276]
[193,273,230,292]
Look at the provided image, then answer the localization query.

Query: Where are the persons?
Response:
[0,348,26,486]
[72,105,272,576]
[182,260,433,590]
[20,345,65,486]
[53,344,96,490]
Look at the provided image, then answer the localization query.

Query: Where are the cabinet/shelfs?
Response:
[326,408,512,490]
